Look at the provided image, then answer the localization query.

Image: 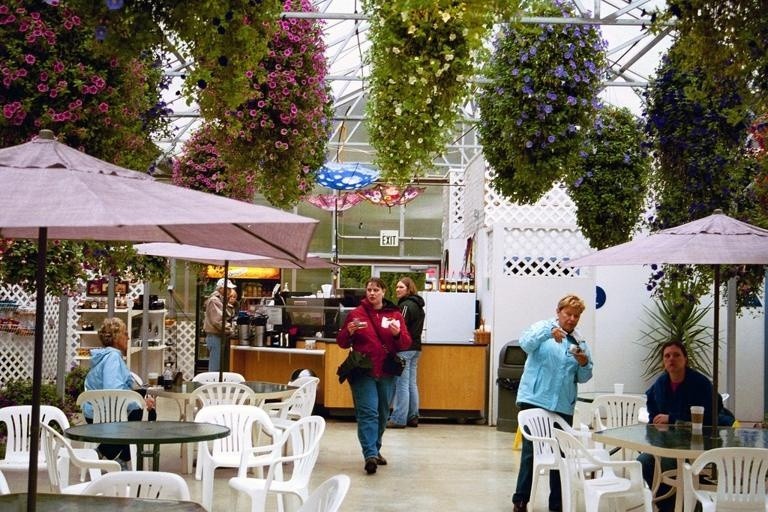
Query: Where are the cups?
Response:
[690,405,705,428]
[147,372,159,385]
[690,428,704,450]
[613,383,625,394]
[304,339,316,351]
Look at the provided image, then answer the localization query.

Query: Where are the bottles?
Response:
[162,363,175,390]
[147,339,162,346]
[241,281,273,298]
[423,278,475,293]
[284,282,290,291]
[82,320,94,328]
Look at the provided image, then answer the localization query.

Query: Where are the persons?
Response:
[81,315,158,470]
[632,340,723,511]
[222,289,237,372]
[204,279,235,374]
[337,278,412,472]
[512,294,591,511]
[384,276,425,429]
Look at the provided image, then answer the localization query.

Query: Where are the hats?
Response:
[216,278,237,288]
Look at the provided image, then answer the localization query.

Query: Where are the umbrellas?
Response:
[305,190,361,213]
[313,161,382,191]
[560,207,767,478]
[112,241,343,391]
[0,129,317,512]
[360,184,427,212]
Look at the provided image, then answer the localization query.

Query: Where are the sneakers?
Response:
[385,421,406,428]
[365,458,377,475]
[378,454,387,465]
[407,417,418,427]
[513,502,528,512]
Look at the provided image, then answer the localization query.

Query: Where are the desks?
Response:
[59,420,230,498]
[144,382,301,480]
[590,421,768,512]
[576,391,648,403]
[0,490,206,511]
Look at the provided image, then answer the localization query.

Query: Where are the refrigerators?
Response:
[194,266,284,378]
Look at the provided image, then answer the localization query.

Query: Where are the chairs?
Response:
[267,375,319,446]
[186,384,259,474]
[0,405,71,495]
[517,407,605,512]
[72,388,150,483]
[192,404,286,512]
[190,372,246,386]
[721,392,730,409]
[587,394,649,431]
[681,446,768,512]
[551,426,653,512]
[225,413,327,512]
[298,473,353,510]
[38,422,121,498]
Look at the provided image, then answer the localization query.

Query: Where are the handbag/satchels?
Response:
[382,350,406,377]
[335,350,375,386]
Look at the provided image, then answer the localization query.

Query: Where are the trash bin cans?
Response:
[496,340,528,432]
